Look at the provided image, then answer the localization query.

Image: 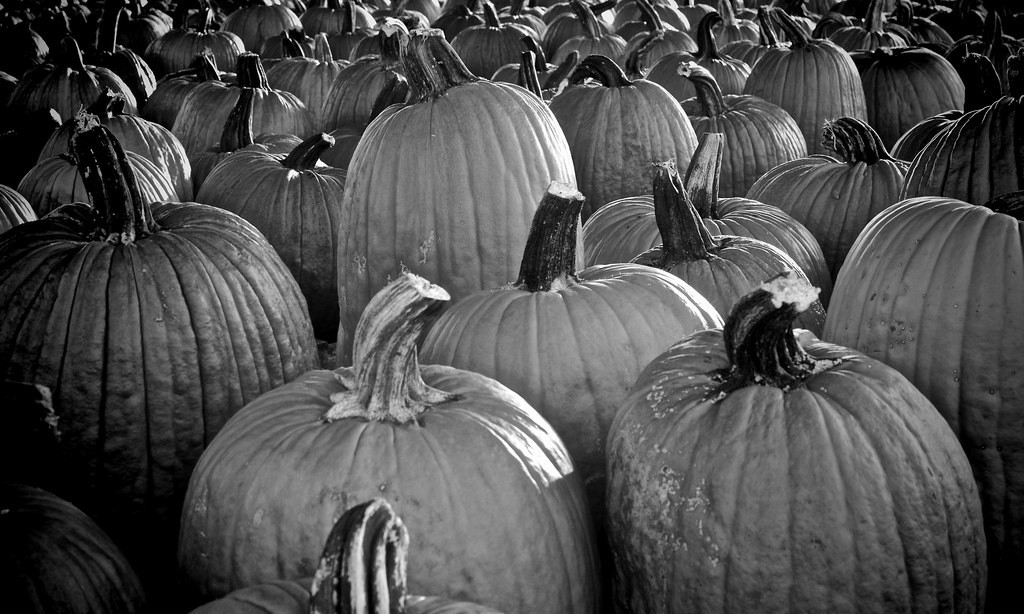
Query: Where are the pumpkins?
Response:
[0,0,1024,614]
